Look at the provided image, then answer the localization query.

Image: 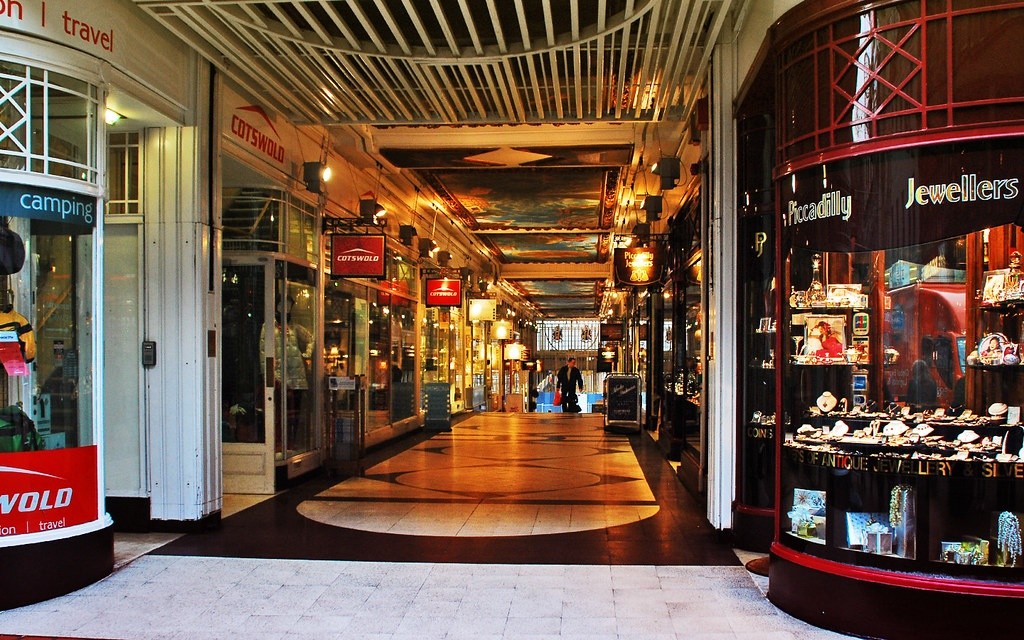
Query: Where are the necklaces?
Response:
[794,392,1009,446]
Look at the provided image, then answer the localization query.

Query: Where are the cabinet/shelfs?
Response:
[956,297,1024,375]
[750,329,776,371]
[790,305,872,366]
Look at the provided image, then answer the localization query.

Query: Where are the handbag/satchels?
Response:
[0,216,41,452]
[553,392,561,406]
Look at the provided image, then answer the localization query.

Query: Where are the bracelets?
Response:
[810,330,814,333]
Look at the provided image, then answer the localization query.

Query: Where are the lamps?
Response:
[640,195,662,221]
[418,237,440,259]
[437,252,454,267]
[631,224,650,243]
[303,159,332,193]
[460,266,541,332]
[360,197,386,226]
[650,157,681,189]
[400,223,418,246]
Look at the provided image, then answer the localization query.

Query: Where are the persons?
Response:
[259,293,318,461]
[855,341,869,362]
[556,359,584,414]
[982,337,1004,359]
[809,322,844,359]
